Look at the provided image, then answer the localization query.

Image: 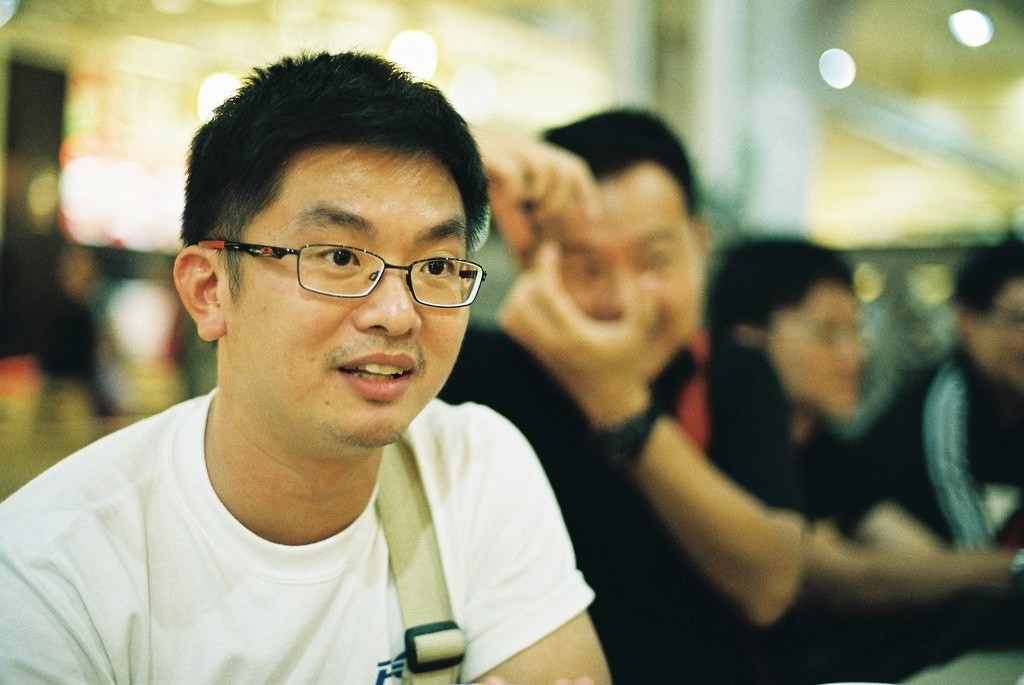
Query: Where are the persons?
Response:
[0,48,619,685]
[710,238,1024,685]
[446,105,817,685]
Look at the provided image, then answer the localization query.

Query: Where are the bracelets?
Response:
[596,399,663,489]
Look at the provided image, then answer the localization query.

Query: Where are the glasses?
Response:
[197,240,486,307]
